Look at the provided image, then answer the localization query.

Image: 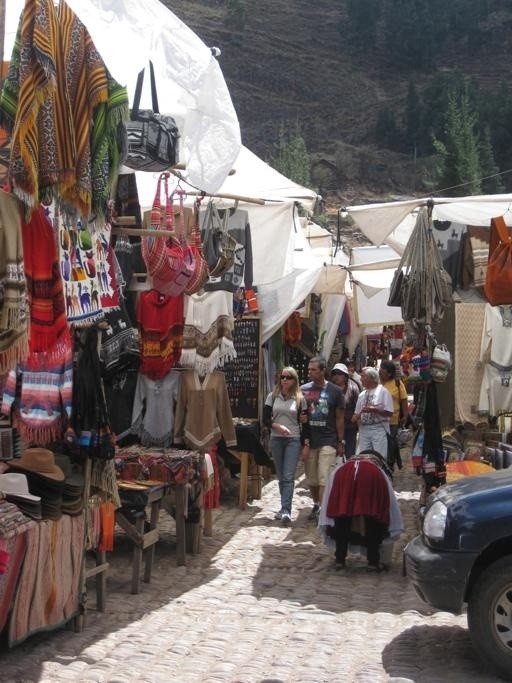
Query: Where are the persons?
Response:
[264,348,413,523]
[317,450,405,571]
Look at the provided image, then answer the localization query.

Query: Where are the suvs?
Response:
[398,461,512,680]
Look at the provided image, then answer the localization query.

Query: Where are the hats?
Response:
[329,362,349,377]
[0,448,86,520]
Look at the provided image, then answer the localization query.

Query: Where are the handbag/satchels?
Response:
[388,433,396,466]
[122,109,179,171]
[484,216,512,306]
[397,428,414,449]
[142,222,237,296]
[386,265,454,324]
[431,344,452,383]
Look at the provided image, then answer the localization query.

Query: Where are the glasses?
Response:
[280,375,295,380]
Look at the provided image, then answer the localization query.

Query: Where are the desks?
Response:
[0,419,261,634]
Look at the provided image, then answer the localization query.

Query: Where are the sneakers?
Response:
[274,506,293,523]
[308,504,319,520]
[366,559,390,572]
